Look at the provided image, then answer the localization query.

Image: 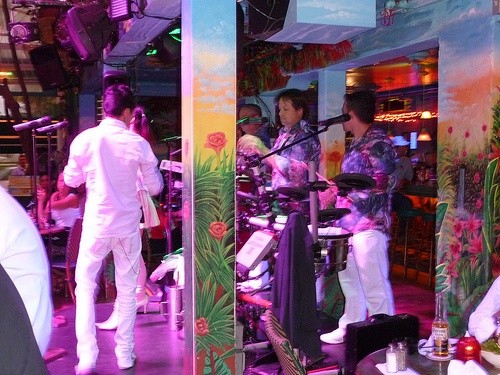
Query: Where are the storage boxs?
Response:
[9,175,41,196]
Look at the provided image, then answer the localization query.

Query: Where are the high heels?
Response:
[95,309,120,330]
[135,295,149,314]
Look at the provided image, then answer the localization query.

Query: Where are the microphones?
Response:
[12,116,52,131]
[162,136,178,142]
[307,114,351,127]
[36,121,70,133]
[240,117,268,125]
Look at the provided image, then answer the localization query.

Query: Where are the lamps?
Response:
[8,21,40,45]
[415,70,434,142]
[383,77,395,140]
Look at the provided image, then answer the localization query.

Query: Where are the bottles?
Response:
[455,331,482,364]
[397,341,407,372]
[433,293,450,358]
[386,344,399,373]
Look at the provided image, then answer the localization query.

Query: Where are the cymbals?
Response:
[276,184,311,202]
[331,171,377,192]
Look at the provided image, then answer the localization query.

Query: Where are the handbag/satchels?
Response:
[344,313,420,375]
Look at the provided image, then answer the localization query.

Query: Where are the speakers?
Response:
[343,315,421,375]
[28,44,69,92]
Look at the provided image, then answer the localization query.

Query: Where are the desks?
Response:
[354,341,500,375]
[236,287,273,367]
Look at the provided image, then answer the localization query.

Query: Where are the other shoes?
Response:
[117,354,135,369]
[319,328,346,344]
[72,363,95,375]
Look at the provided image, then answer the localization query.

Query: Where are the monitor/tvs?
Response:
[65,4,114,62]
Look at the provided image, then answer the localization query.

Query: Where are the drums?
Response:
[308,226,353,276]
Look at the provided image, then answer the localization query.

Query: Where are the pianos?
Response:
[159,158,184,178]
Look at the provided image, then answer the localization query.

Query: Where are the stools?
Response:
[414,213,436,289]
[388,206,425,282]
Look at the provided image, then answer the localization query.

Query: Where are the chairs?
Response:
[343,313,422,375]
[50,217,108,304]
[262,307,342,375]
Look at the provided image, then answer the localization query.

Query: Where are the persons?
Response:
[392,145,432,236]
[64,86,164,375]
[9,153,29,181]
[28,169,81,310]
[237,90,321,211]
[320,90,400,354]
[235,103,270,153]
[469,275,500,344]
